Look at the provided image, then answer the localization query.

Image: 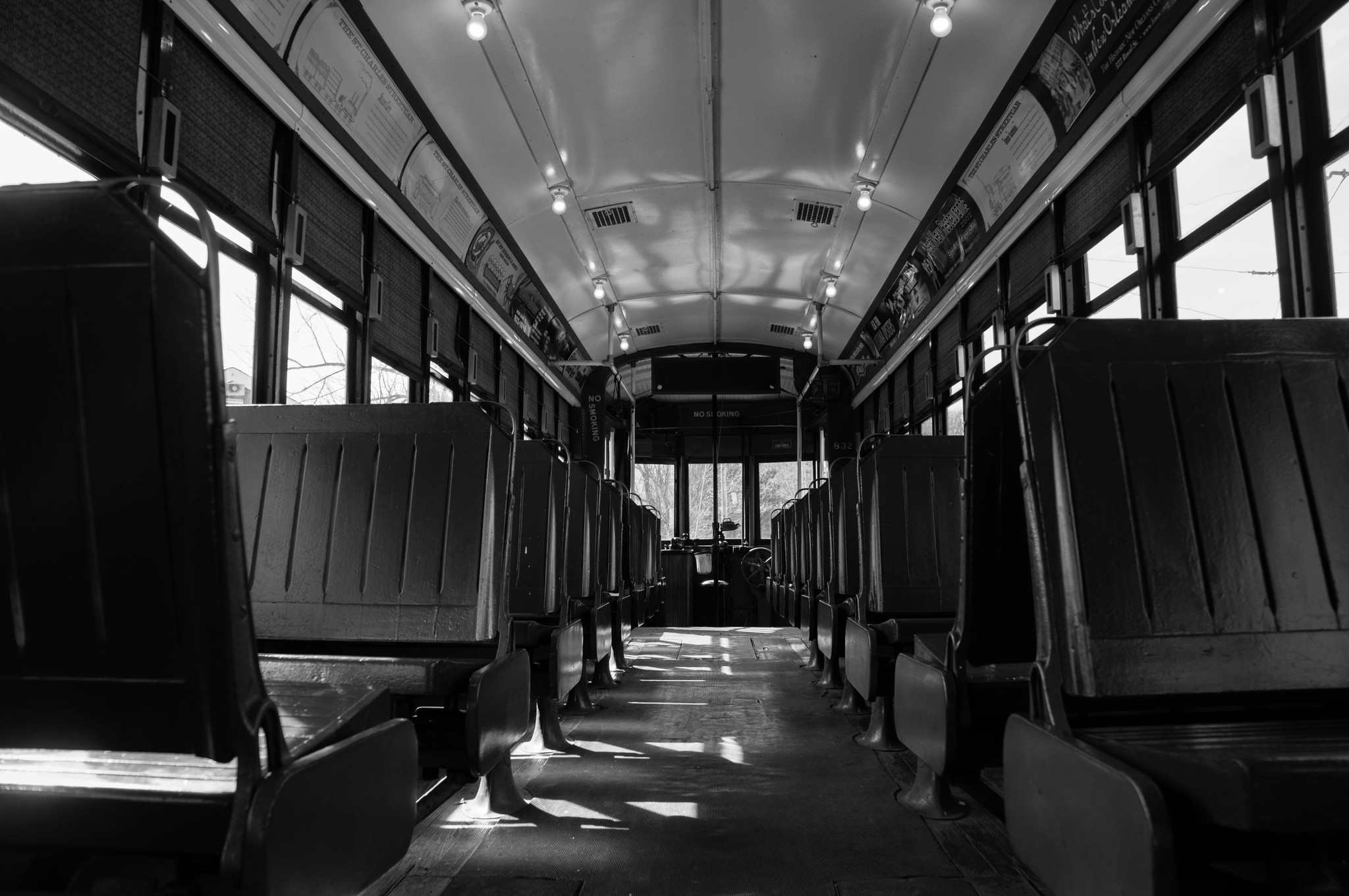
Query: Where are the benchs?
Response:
[0,177,665,896]
[766,311,1349,896]
